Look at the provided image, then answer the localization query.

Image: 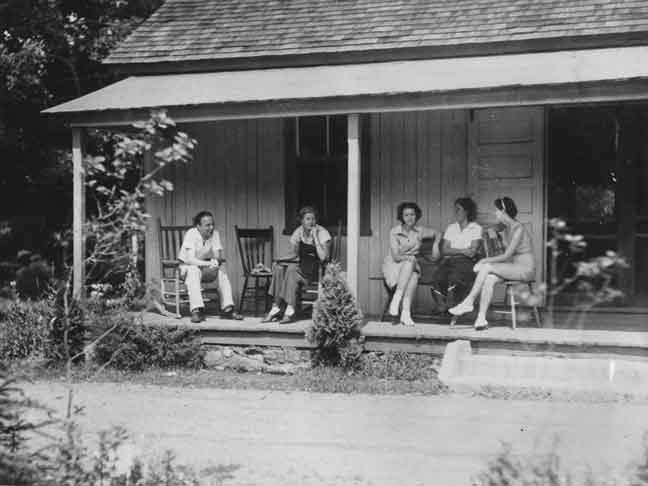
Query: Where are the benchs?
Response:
[368,235,482,328]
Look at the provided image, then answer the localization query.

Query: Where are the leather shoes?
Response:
[279,311,297,323]
[261,310,282,322]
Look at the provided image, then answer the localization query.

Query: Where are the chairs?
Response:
[483,229,541,328]
[153,217,220,318]
[233,224,277,315]
[282,221,345,320]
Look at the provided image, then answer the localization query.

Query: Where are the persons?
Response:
[261,204,332,325]
[569,171,617,225]
[176,211,244,325]
[447,196,538,332]
[428,196,483,317]
[14,247,50,304]
[380,201,445,327]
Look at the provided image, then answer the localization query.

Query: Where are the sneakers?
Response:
[224,309,244,320]
[191,309,202,323]
[475,318,489,330]
[399,309,415,326]
[447,303,474,317]
[388,297,401,317]
